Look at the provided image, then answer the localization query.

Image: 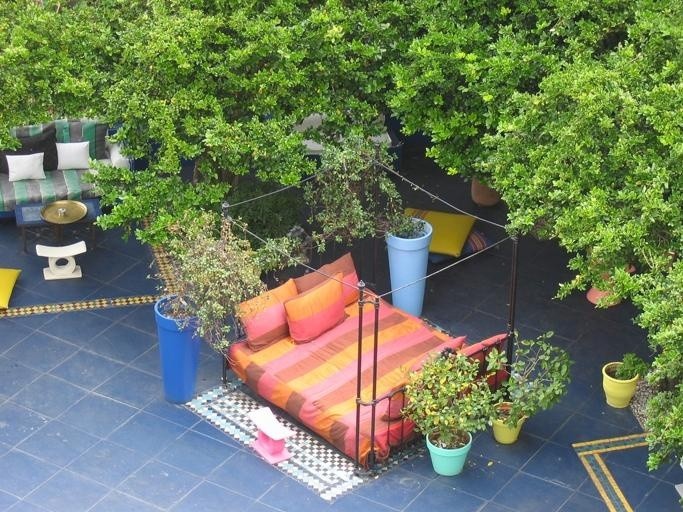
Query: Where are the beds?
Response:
[220,157,518,480]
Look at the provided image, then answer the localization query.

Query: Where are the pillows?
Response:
[404,206,477,258]
[6,151,46,181]
[380,332,510,421]
[400,334,468,376]
[428,225,498,265]
[0,128,58,175]
[56,141,90,169]
[235,278,299,352]
[284,270,346,343]
[0,263,22,309]
[294,251,368,306]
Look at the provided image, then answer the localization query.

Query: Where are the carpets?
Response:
[183,316,546,505]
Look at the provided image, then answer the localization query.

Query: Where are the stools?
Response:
[36,240,87,280]
[245,407,294,467]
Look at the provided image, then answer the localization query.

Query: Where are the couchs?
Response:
[0,120,135,219]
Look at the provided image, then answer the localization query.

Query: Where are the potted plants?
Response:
[304,138,432,319]
[601,353,649,409]
[400,347,506,478]
[146,209,302,406]
[479,329,577,446]
